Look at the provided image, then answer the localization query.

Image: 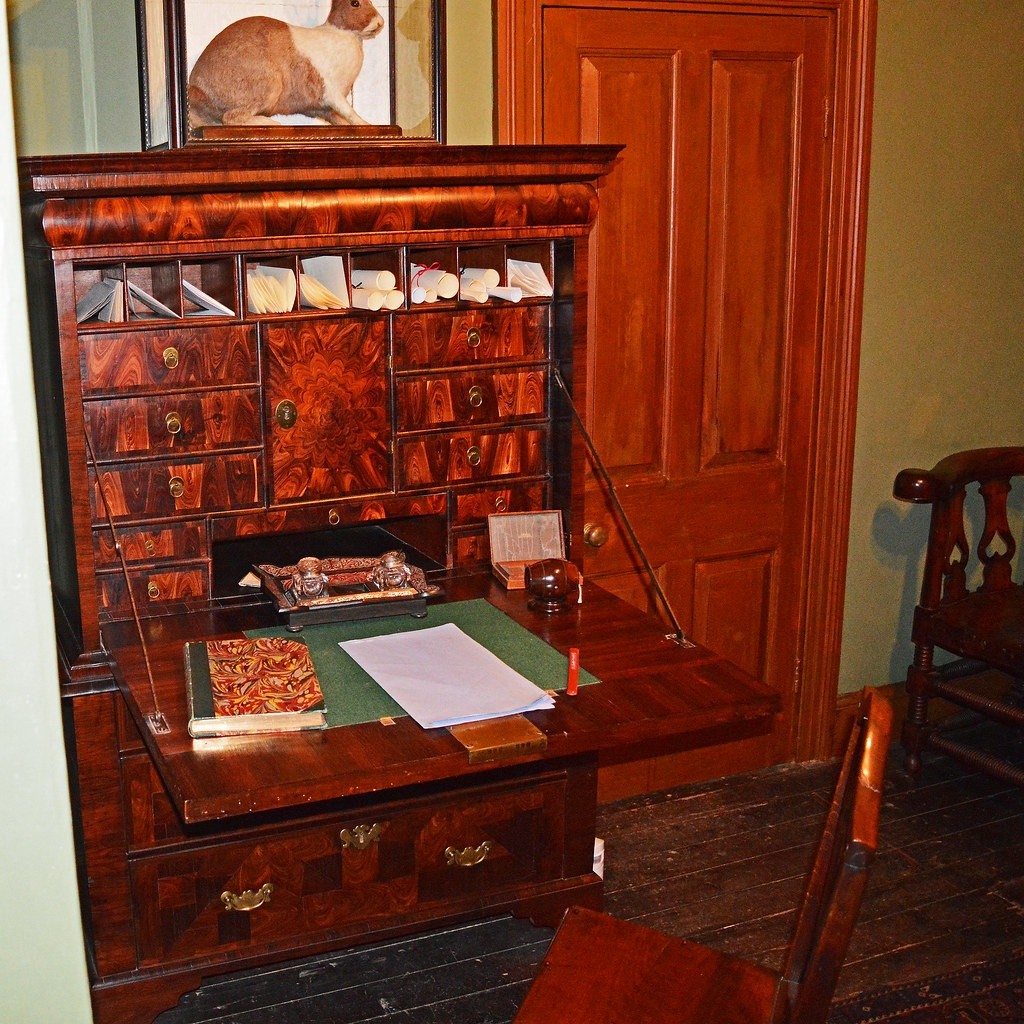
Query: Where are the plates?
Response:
[252,547,439,612]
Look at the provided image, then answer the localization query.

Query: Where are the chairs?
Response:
[891,446,1024,791]
[513,683,893,1024]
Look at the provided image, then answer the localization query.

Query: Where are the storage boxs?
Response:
[488,509,583,590]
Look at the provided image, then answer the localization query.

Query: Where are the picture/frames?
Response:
[134,0,447,152]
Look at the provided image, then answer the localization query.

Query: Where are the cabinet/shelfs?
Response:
[15,144,780,1024]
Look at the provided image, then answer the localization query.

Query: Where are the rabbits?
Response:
[188,0,386,130]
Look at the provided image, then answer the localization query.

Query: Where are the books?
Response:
[183,636,328,738]
